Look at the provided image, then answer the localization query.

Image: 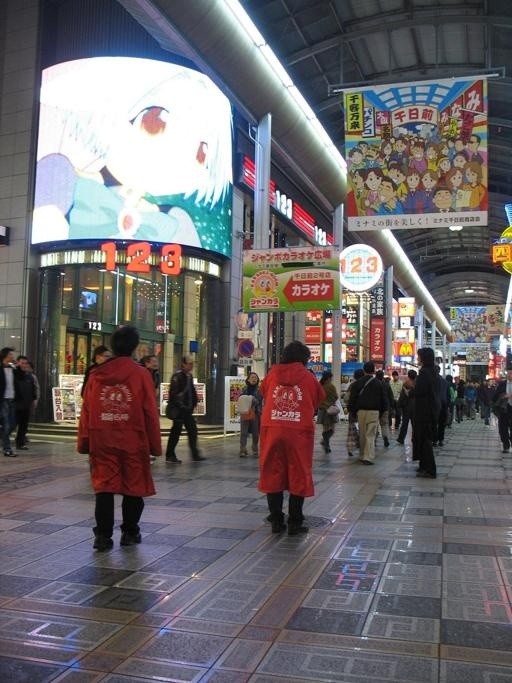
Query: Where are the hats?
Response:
[90,346,109,361]
[180,355,195,364]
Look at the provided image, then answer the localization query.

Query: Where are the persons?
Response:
[80,343,111,396]
[76,323,163,550]
[315,359,512,464]
[142,352,161,465]
[259,338,327,535]
[164,354,211,464]
[12,354,30,449]
[235,371,262,458]
[0,345,24,455]
[26,361,41,443]
[402,346,442,478]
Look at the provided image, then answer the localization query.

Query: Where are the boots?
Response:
[321,429,334,453]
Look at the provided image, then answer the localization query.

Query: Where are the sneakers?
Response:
[93,535,114,550]
[270,521,286,536]
[239,447,258,458]
[120,531,141,545]
[166,454,209,462]
[360,458,374,466]
[4,437,30,458]
[383,435,390,449]
[287,523,308,535]
[415,468,436,479]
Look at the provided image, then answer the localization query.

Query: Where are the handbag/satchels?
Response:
[166,398,185,419]
[326,404,340,416]
